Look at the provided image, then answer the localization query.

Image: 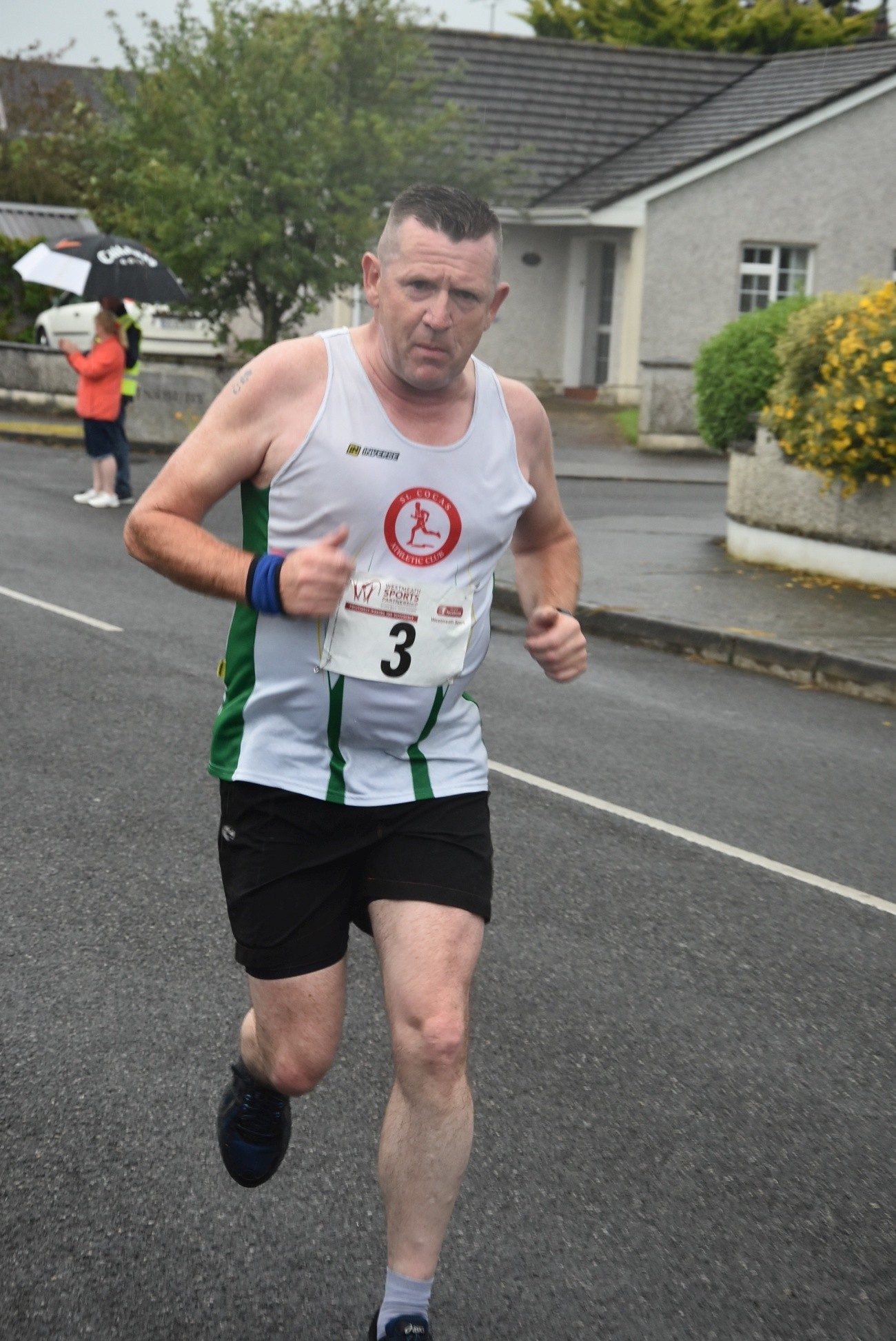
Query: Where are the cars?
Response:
[33,288,238,373]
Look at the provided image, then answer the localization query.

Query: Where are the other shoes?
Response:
[73,489,119,508]
[117,492,135,505]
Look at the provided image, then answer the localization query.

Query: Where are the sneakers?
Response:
[368,1306,433,1341]
[216,1053,293,1187]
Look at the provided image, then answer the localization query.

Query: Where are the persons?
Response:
[78,293,142,503]
[58,312,125,508]
[120,180,589,1340]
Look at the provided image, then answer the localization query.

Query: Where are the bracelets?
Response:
[244,550,294,619]
[554,608,573,616]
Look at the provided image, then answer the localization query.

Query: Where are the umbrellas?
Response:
[10,232,192,304]
